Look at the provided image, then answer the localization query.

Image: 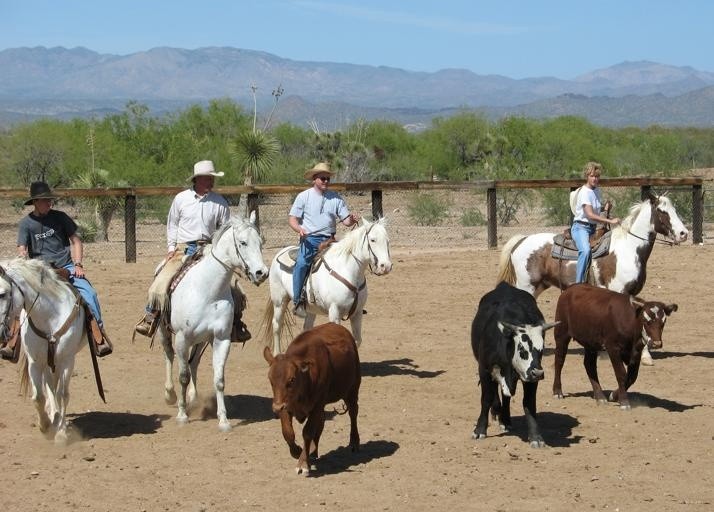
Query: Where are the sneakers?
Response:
[96,336,109,354]
[0,342,20,358]
[135,319,150,335]
[295,304,307,317]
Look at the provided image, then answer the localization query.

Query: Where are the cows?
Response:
[552,283,677,410]
[470,280,562,448]
[263,322,361,476]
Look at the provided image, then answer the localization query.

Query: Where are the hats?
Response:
[303,163,336,180]
[185,161,224,184]
[20,182,57,206]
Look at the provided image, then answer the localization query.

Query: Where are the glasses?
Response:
[313,176,330,182]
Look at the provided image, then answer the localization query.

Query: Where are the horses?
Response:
[244,213,393,357]
[155,210,268,431]
[495,190,688,365]
[0,255,88,446]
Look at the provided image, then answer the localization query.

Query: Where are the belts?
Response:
[573,220,596,228]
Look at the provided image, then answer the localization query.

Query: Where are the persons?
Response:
[570,161,621,284]
[287,161,359,318]
[136,159,252,342]
[0,181,111,363]
